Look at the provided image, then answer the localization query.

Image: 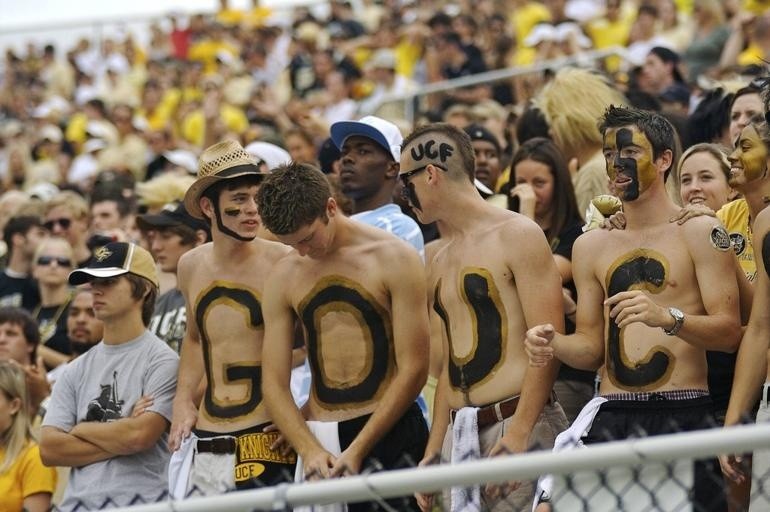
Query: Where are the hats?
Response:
[330,115,404,168]
[465,122,501,153]
[183,137,272,219]
[137,199,212,242]
[67,242,161,288]
[245,137,292,170]
[162,148,197,173]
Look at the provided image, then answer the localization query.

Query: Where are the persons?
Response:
[0,1,770,512]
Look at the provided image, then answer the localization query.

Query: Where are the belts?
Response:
[448,396,519,430]
[188,436,237,455]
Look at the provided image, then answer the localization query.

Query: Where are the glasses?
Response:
[37,256,71,269]
[749,74,769,93]
[41,218,72,229]
[397,162,448,188]
